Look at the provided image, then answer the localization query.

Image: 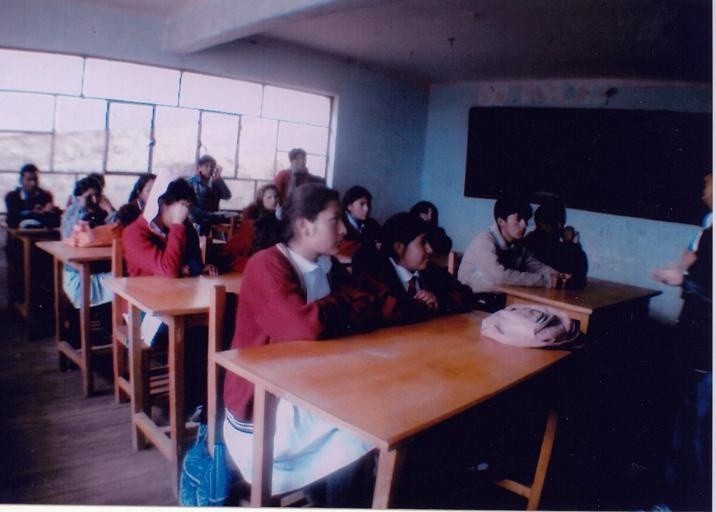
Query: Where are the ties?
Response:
[407,277,419,298]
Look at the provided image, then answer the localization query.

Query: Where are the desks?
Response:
[101,275,244,498]
[212,310,572,511]
[209,223,240,239]
[35,238,226,399]
[5,227,60,318]
[491,277,663,335]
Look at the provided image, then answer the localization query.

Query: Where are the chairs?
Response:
[111,239,168,404]
[207,285,311,507]
[229,215,240,239]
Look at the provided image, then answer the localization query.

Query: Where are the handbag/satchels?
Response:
[178,290,237,507]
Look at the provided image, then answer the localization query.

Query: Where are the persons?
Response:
[222,184,377,502]
[363,212,477,328]
[120,167,220,421]
[522,200,589,286]
[243,184,281,215]
[58,177,118,311]
[273,148,308,206]
[3,165,55,227]
[222,219,264,273]
[409,200,439,222]
[456,194,570,296]
[650,170,712,375]
[129,173,157,210]
[89,173,116,214]
[183,154,231,236]
